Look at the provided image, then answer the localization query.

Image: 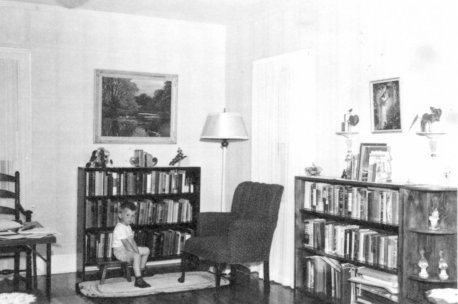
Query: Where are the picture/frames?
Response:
[91,66,179,145]
[358,143,386,180]
[369,76,402,133]
[372,155,392,184]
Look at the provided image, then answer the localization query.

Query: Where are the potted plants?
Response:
[86,147,113,166]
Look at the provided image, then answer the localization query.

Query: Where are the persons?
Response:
[111,201,151,288]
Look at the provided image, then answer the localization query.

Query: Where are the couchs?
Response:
[176,181,284,304]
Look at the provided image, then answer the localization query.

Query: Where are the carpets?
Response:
[0,288,50,304]
[75,268,233,299]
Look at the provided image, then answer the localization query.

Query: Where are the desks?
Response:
[0,221,56,302]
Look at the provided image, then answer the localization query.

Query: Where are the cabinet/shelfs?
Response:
[293,176,458,304]
[76,166,201,280]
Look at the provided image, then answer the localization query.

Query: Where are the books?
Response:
[302,181,400,304]
[86,170,194,262]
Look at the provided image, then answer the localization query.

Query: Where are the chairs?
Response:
[0,171,33,289]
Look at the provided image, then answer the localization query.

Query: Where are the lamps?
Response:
[199,107,249,214]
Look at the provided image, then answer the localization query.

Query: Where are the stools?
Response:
[99,260,131,285]
